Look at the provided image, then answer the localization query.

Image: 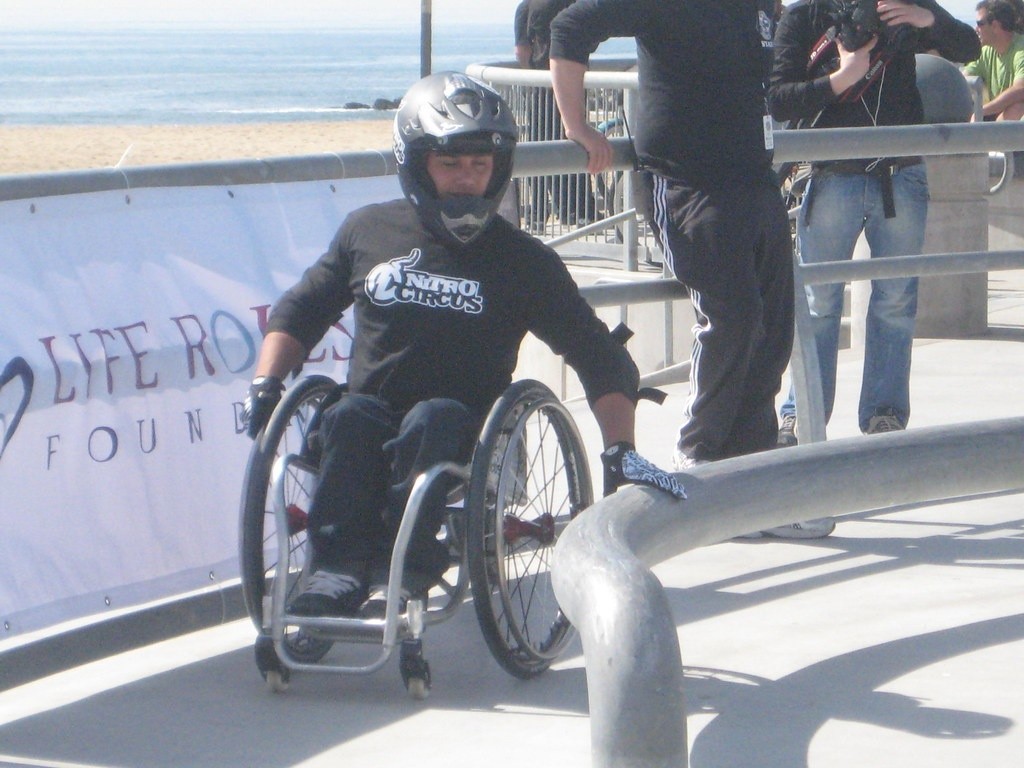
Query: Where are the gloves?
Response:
[600,444,688,501]
[242,376,282,440]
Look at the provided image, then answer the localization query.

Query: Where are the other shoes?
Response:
[867,414,903,433]
[525,211,608,237]
[736,518,836,541]
[777,415,799,447]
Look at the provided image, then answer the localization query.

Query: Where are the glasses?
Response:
[977,19,992,26]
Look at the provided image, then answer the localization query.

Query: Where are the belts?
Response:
[824,156,921,175]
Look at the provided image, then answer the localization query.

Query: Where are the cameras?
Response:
[838,0,891,53]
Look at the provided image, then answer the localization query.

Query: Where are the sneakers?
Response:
[361,584,413,616]
[290,568,367,616]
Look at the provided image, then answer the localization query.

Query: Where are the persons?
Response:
[513,0,1024,241]
[767,1,981,449]
[239,72,688,616]
[552,0,841,541]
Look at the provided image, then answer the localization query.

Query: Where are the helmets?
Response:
[394,71,518,251]
